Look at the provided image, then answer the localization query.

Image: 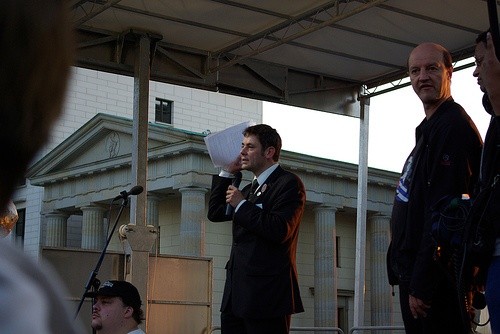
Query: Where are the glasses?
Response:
[476,57,484,66]
[407,66,449,75]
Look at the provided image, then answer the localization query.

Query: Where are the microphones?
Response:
[225,171,242,215]
[112,185,143,201]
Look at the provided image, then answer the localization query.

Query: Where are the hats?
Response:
[84,280,141,298]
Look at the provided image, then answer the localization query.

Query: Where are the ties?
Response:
[248,179,259,202]
